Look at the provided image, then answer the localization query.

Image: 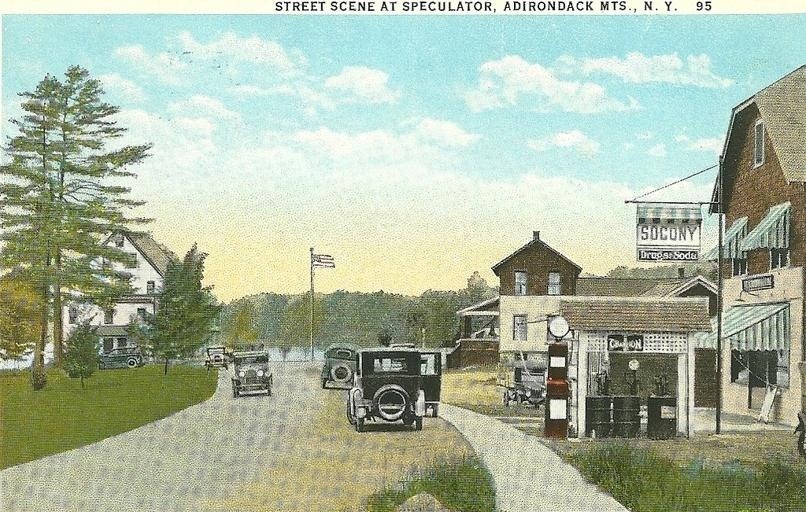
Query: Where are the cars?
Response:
[99,345,145,369]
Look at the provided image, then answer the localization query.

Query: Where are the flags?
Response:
[312,255,336,268]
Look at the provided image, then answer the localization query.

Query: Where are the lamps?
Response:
[734,289,759,301]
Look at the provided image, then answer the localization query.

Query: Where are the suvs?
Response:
[231,350,272,397]
[320,343,441,432]
[205,345,228,370]
[502,361,547,407]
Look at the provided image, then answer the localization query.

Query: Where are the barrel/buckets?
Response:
[648,397,676,439]
[613,397,641,437]
[586,396,611,437]
